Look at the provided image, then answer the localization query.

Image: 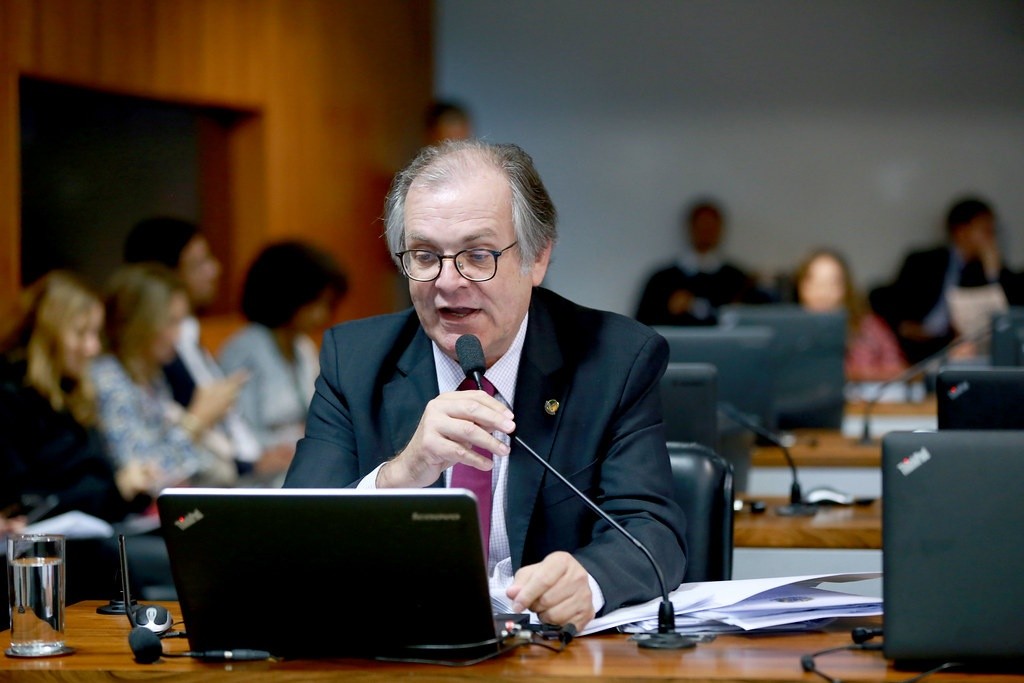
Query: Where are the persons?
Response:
[123,216,296,487]
[222,237,345,487]
[875,195,1024,364]
[0,272,176,601]
[283,139,685,631]
[795,247,903,382]
[634,202,763,327]
[86,261,247,517]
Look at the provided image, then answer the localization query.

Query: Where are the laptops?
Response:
[878,307,1024,676]
[156,487,532,664]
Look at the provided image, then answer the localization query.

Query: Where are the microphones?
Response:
[456,334,697,651]
[94,534,163,666]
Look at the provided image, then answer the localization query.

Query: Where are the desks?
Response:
[0,369,1024,683]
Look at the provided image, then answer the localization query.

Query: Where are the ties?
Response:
[449,377,496,559]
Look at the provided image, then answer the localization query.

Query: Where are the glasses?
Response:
[316,288,346,314]
[394,237,518,282]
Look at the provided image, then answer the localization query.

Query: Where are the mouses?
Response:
[132,605,175,635]
[803,486,856,506]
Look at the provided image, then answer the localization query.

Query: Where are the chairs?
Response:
[664,443,735,584]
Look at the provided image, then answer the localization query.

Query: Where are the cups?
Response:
[7,534,65,653]
